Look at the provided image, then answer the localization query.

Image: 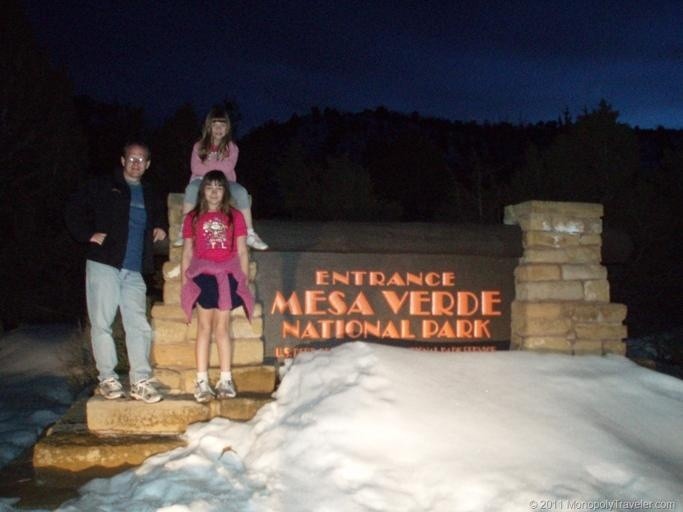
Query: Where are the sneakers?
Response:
[246,228,269,250]
[131,378,164,404]
[215,377,236,400]
[172,228,184,248]
[97,379,126,400]
[192,374,215,403]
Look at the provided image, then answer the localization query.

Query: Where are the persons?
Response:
[173,105,269,253]
[70,138,169,405]
[181,169,255,402]
